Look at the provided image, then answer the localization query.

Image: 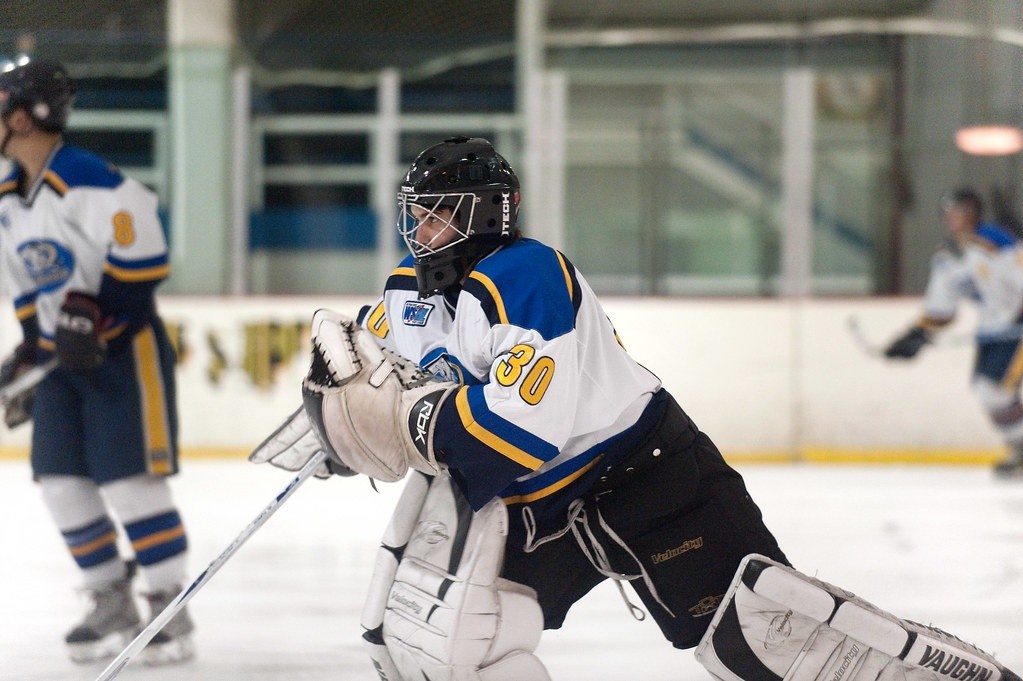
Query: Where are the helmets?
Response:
[0,56,70,128]
[397,137,520,294]
[935,184,982,230]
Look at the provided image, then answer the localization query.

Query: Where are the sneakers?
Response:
[65,563,144,661]
[127,587,194,665]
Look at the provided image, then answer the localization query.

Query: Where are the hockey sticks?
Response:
[0,353,70,403]
[90,447,336,681]
[848,305,1020,366]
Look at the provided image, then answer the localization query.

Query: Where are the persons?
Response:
[0,52,198,664]
[249,139,1023,681]
[885,189,1023,474]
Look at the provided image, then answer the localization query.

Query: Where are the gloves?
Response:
[56,291,105,370]
[0,338,44,428]
[887,326,934,356]
